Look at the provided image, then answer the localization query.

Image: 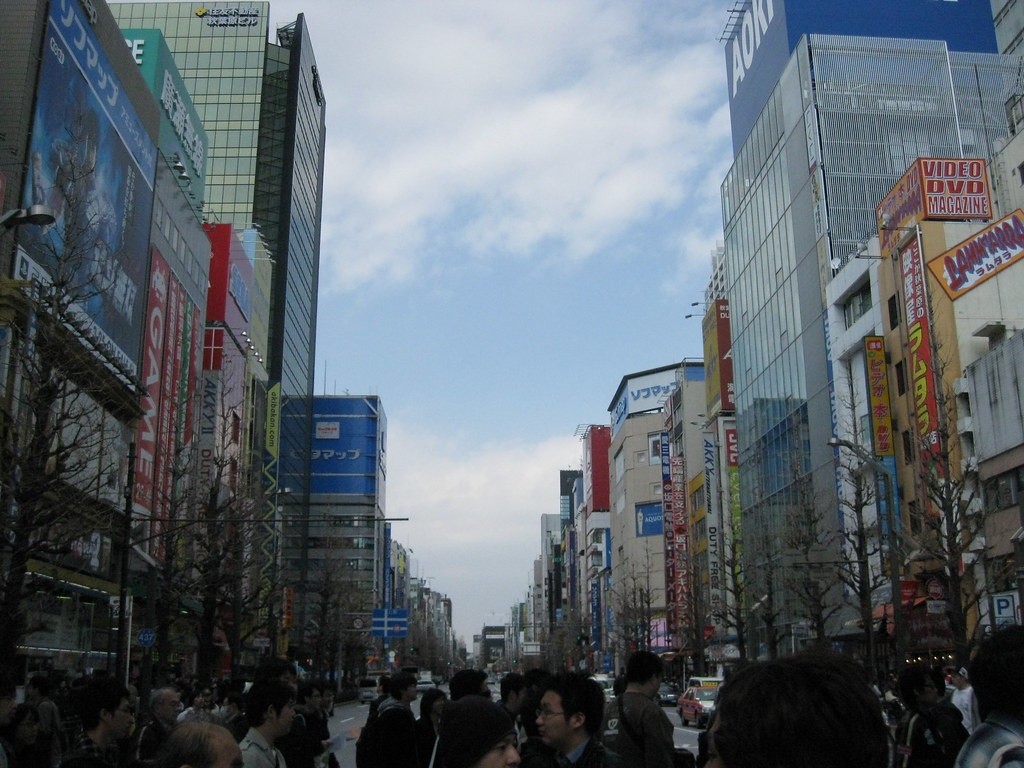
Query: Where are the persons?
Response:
[0,628,1022,767]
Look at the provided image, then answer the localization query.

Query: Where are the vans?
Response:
[688,677,724,690]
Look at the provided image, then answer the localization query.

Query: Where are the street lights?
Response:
[827,436,905,672]
[389,649,396,674]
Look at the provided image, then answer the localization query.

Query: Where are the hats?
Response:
[439,694,518,767]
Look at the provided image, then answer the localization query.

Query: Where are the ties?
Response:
[559,754,569,768]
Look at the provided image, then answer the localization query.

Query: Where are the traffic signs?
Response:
[577,635,581,641]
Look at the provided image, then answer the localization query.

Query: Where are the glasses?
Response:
[18,722,41,731]
[534,709,564,720]
[310,695,322,701]
[323,694,335,698]
[203,693,212,697]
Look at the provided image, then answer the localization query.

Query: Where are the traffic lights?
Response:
[513,659,517,663]
[410,647,414,651]
[358,670,677,705]
[447,661,451,666]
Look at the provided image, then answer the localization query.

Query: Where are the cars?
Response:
[677,687,718,727]
